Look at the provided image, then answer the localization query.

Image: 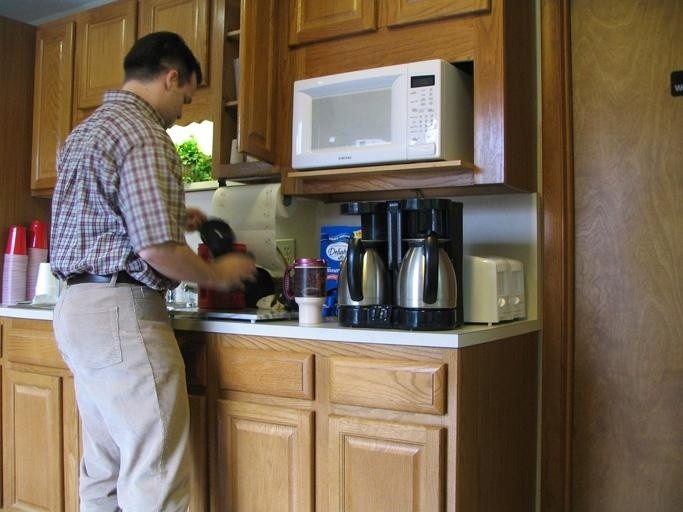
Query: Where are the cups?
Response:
[282,257,327,323]
[2,218,48,307]
[34,262,57,296]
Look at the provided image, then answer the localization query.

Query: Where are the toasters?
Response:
[463,254,526,324]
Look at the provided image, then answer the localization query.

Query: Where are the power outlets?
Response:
[277,238,297,267]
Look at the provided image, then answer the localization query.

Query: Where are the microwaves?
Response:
[291,59,467,171]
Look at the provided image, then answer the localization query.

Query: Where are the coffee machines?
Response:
[335,199,465,330]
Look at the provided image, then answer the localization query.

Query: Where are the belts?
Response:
[65,271,147,286]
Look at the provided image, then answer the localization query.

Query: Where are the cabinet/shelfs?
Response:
[1,303,207,512]
[30,13,76,198]
[76,0,211,123]
[209,316,537,512]
[210,0,273,184]
[278,0,535,204]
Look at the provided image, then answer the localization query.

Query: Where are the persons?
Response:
[48,30,259,512]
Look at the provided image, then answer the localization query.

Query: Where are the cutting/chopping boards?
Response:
[202,310,299,321]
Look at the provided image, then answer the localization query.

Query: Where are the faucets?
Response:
[184,282,199,295]
